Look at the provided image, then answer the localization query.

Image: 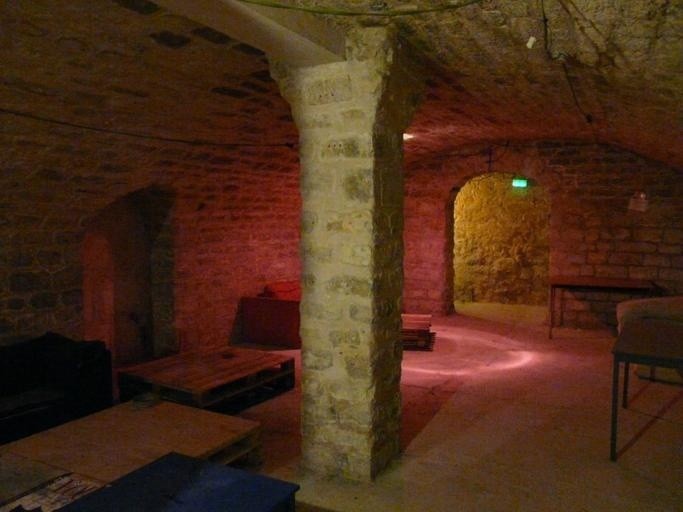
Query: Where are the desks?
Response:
[50,450,302,511]
[4,394,263,489]
[241,291,301,349]
[547,273,657,338]
[608,317,682,458]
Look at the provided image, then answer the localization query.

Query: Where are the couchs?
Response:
[0,329,118,443]
